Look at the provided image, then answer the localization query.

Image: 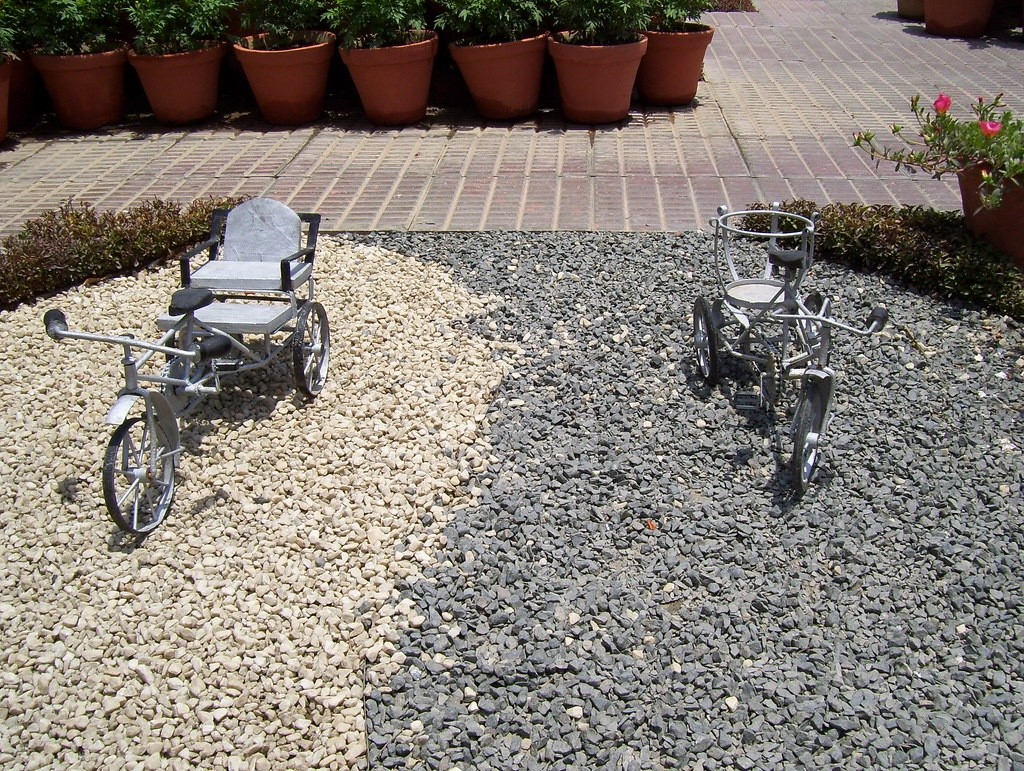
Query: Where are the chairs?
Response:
[179,197,321,315]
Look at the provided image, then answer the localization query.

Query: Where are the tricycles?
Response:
[44,197,331,539]
[692,201,890,495]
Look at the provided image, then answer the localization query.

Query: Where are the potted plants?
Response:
[0,0,24,144]
[319,0,439,128]
[635,0,714,107]
[433,0,551,122]
[26,0,130,130]
[231,0,336,125]
[546,0,648,125]
[124,0,227,124]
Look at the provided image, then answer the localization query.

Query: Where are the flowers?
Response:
[851,92,1024,209]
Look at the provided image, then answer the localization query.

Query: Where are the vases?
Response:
[925,0,991,38]
[957,167,1024,261]
[897,0,925,21]
